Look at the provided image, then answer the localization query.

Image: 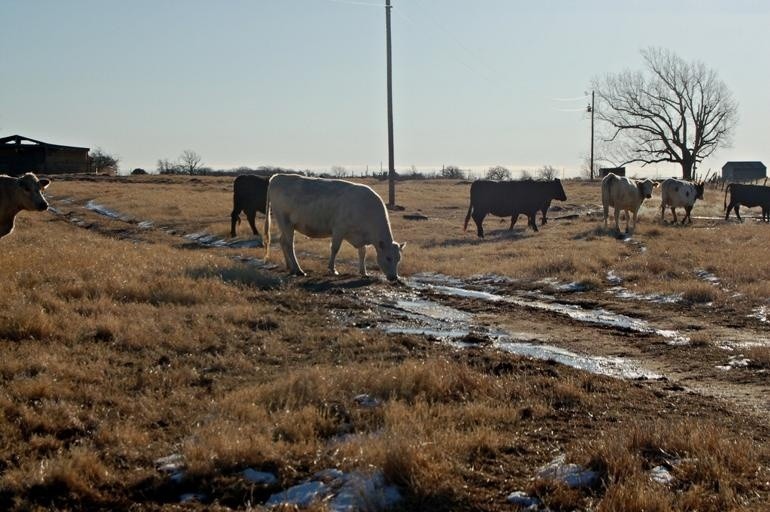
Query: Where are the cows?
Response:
[601,172,659,233]
[723,182,770,223]
[661,178,705,223]
[463,177,568,239]
[262,172,407,282]
[230,174,272,238]
[0,172,51,239]
